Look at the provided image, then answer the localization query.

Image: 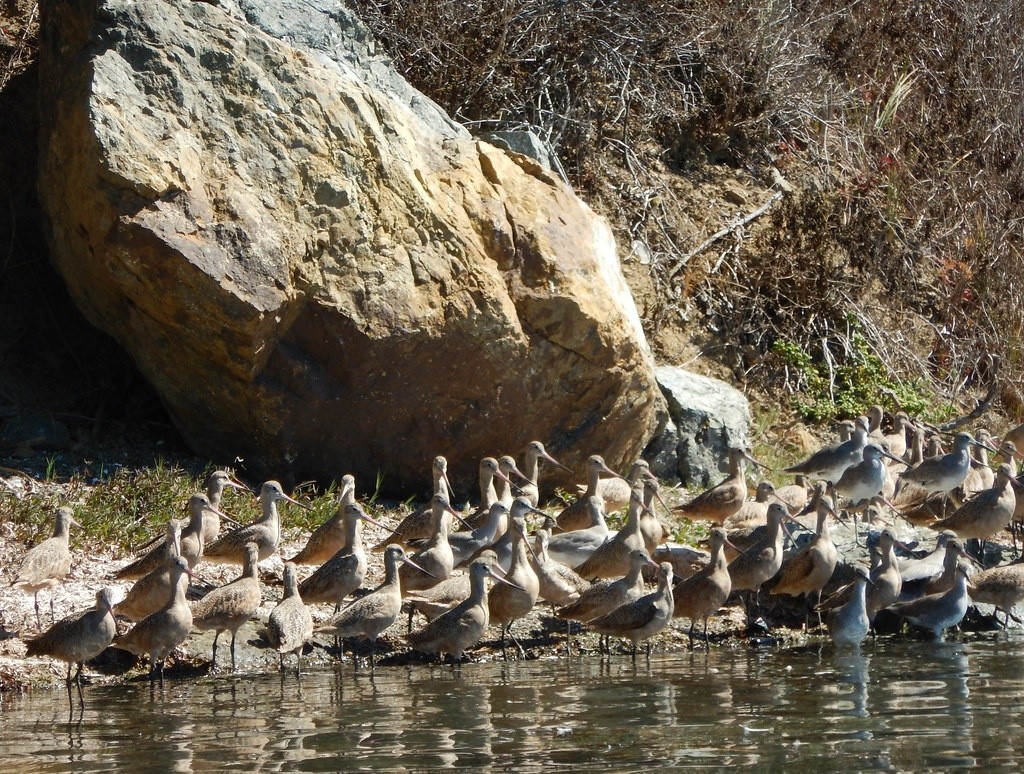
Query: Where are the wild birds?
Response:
[7,506,86,632]
[109,402,1024,693]
[19,586,121,712]
